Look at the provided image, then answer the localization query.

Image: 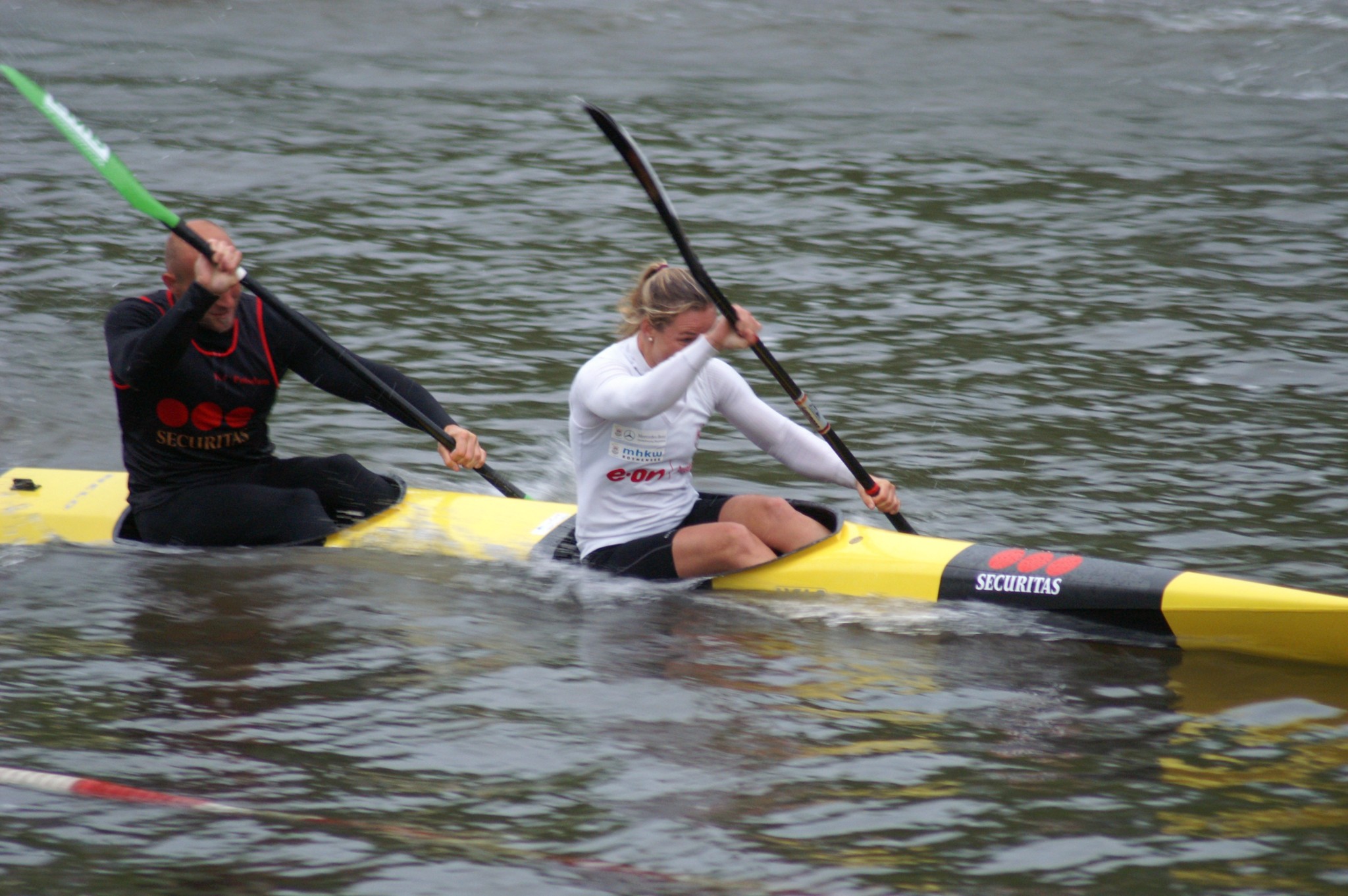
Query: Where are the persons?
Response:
[567,253,902,585]
[103,218,488,551]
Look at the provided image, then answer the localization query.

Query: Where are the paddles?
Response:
[570,89,917,540]
[0,62,529,503]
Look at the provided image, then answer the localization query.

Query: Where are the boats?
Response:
[0,467,1348,666]
